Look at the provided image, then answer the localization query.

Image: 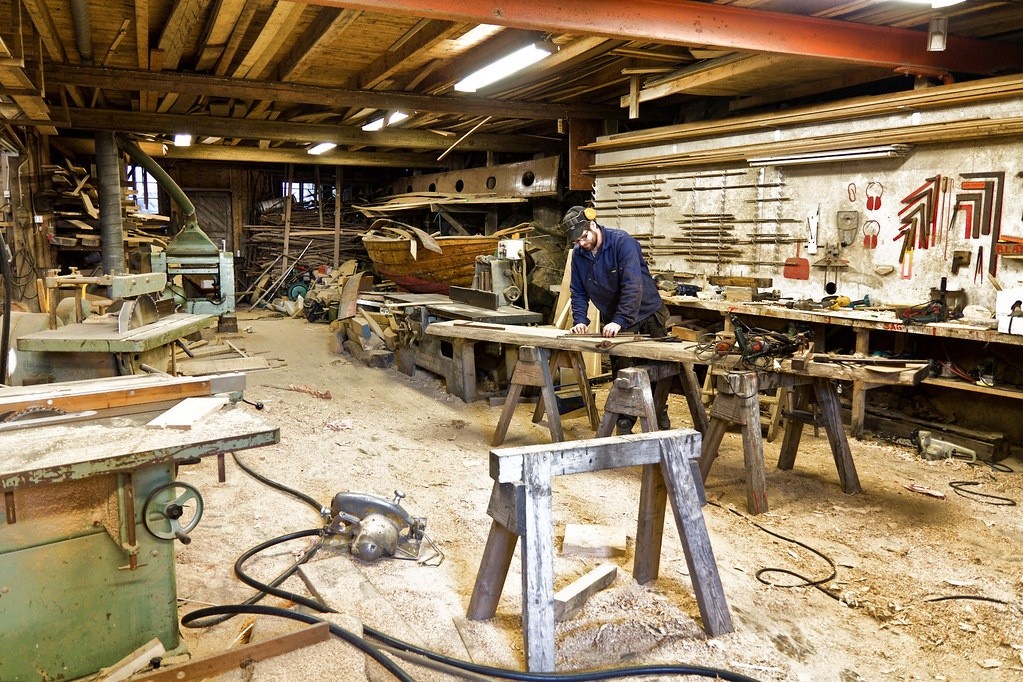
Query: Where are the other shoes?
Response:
[614,420,633,436]
[658,405,671,429]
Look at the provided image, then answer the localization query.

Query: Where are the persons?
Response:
[562,206,670,435]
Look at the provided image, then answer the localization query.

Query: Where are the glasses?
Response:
[572,229,589,244]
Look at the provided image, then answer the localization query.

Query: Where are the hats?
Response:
[561,206,596,243]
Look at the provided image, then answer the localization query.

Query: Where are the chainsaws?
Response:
[323,489,446,567]
[910,428,976,463]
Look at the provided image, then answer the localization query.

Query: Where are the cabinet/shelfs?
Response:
[656,291,1023,474]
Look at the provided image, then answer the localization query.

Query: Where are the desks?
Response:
[427,316,933,515]
[385,295,543,402]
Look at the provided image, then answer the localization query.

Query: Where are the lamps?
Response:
[306,109,414,156]
[455,38,561,95]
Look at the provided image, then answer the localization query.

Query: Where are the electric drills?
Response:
[773,295,850,311]
